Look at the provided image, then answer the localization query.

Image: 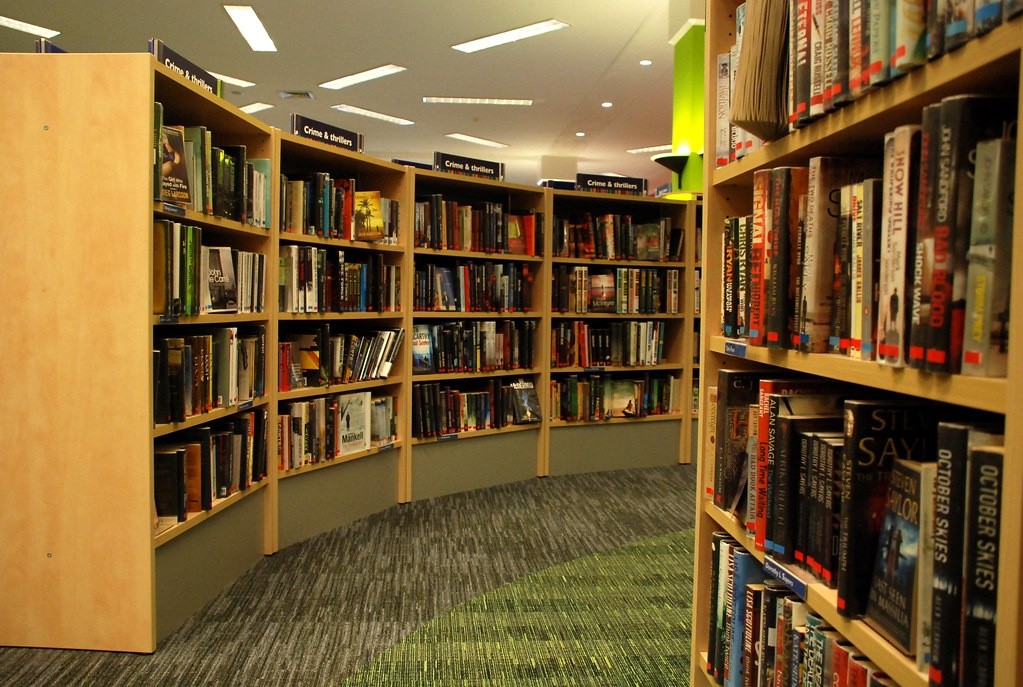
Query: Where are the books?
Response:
[706,0,1023,687]
[147,100,703,523]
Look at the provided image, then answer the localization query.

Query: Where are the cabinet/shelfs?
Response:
[0,1,1023,687]
